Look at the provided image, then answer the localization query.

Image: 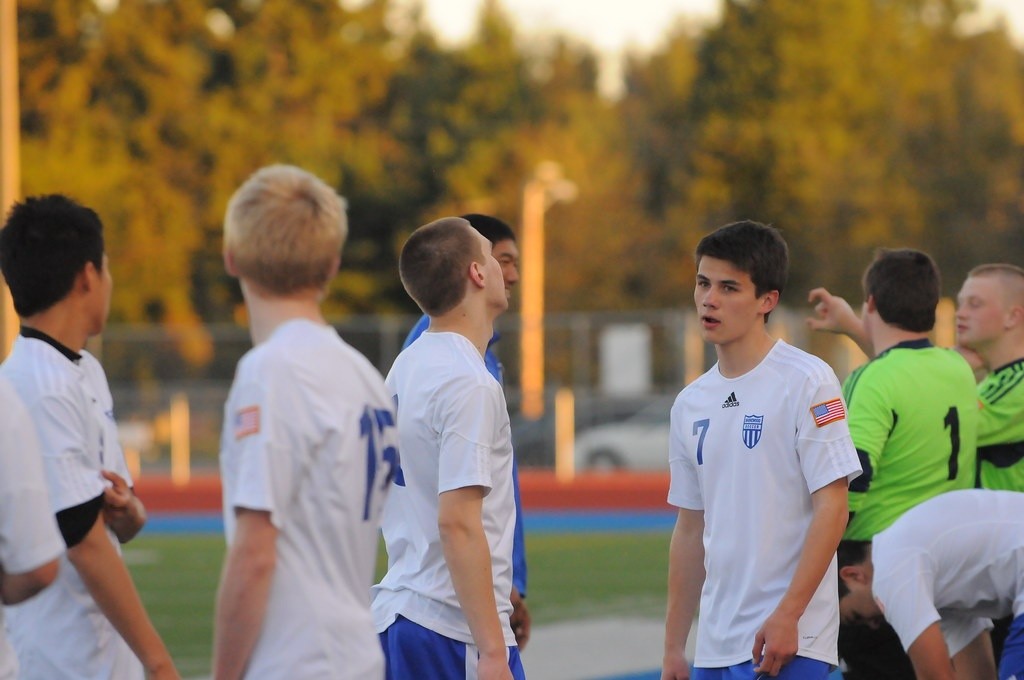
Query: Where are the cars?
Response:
[573,396,678,474]
[509,392,650,473]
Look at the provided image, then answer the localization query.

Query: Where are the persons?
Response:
[0,193,180,680]
[370,213,531,680]
[837,488,1024,680]
[661,219,863,680]
[807,249,978,680]
[956,266,1024,680]
[212,166,398,680]
